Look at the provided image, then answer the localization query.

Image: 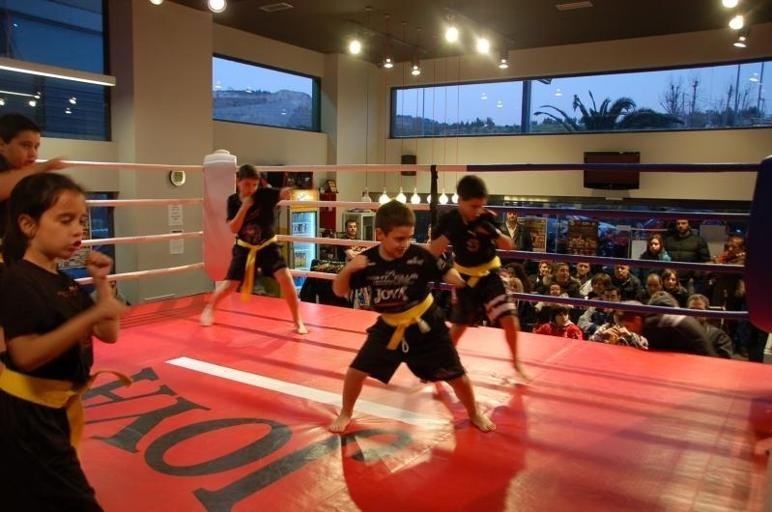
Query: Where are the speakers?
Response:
[401,155,416,176]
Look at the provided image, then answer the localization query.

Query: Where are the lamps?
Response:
[360,51,460,206]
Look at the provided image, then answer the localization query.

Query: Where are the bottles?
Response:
[290,211,315,285]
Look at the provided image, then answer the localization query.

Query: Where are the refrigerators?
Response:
[273,187,321,296]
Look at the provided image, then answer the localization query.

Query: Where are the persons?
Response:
[744,156,772,333]
[0,172,124,512]
[1,110,71,356]
[500,209,769,361]
[338,220,367,262]
[199,164,309,335]
[330,200,497,433]
[420,174,534,383]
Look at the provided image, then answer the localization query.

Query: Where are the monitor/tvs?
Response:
[583,151,640,191]
